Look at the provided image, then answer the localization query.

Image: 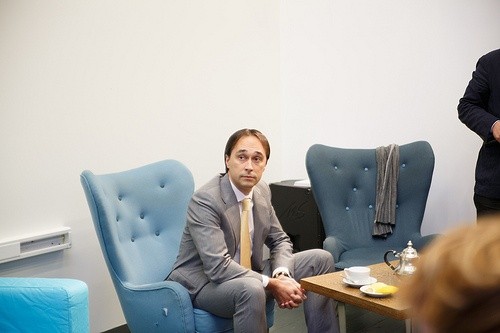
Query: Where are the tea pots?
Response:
[383,240,420,282]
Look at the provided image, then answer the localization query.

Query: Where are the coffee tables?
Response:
[300,259,417,333]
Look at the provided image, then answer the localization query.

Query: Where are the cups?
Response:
[343,266,370,284]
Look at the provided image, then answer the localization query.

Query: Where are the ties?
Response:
[240,198,251,269]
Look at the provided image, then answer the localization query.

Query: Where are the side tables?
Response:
[269,179,326,253]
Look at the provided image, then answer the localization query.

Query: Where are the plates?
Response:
[344,277,378,288]
[359,283,393,298]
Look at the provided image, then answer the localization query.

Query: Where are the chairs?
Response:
[305,141,442,271]
[80,158,274,333]
[0,276,90,333]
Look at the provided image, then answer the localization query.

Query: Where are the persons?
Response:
[165,129,339,333]
[396,216,500,333]
[458,50,500,220]
